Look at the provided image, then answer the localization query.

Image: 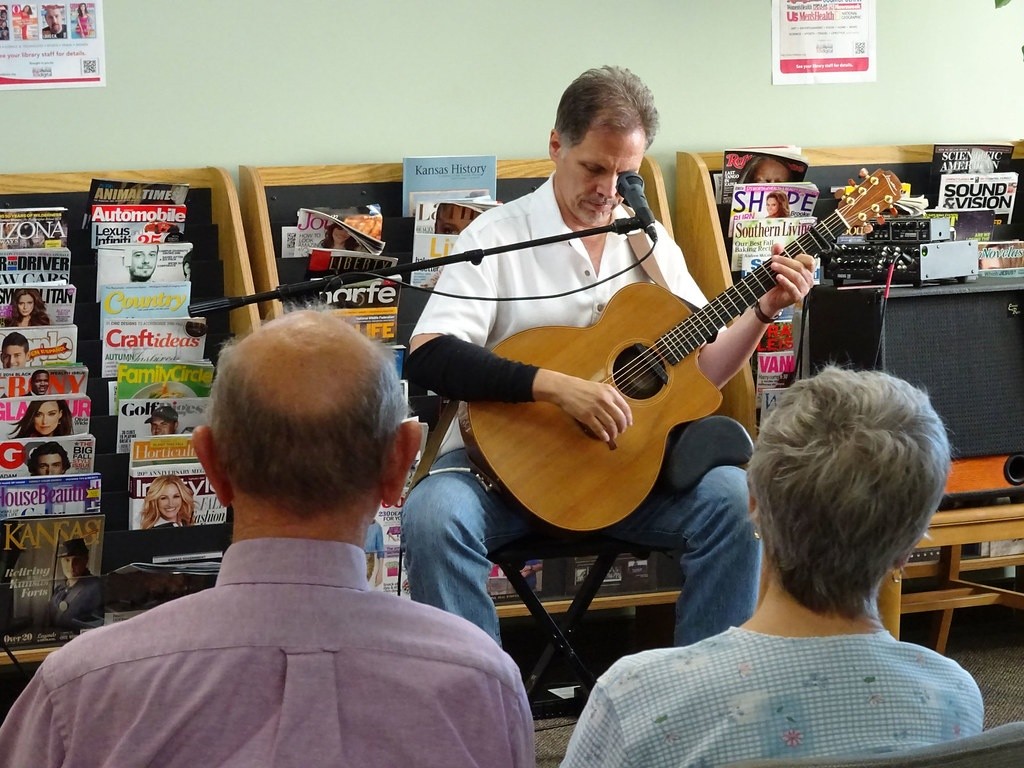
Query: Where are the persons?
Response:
[5,288,50,328]
[7,398,74,439]
[556,363,990,767]
[42,536,106,638]
[15,4,34,40]
[0,331,31,370]
[138,474,196,529]
[402,60,817,707]
[20,370,50,397]
[27,442,71,478]
[42,6,68,40]
[75,3,93,39]
[0,4,10,41]
[0,312,535,768]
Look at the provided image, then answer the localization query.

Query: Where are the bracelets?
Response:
[750,295,786,325]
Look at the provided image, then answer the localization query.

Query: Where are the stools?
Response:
[483,532,660,728]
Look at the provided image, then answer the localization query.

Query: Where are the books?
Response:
[0,323,79,370]
[0,205,68,253]
[0,363,89,402]
[83,173,217,451]
[283,154,544,623]
[0,245,71,290]
[127,429,231,529]
[0,393,93,446]
[0,279,77,328]
[1,472,104,526]
[709,118,1024,453]
[0,432,97,486]
[0,512,110,650]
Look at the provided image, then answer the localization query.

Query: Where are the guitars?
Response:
[456,161,907,536]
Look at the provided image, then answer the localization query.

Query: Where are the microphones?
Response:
[616,171,658,242]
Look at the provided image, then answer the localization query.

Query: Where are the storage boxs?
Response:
[757,503,1023,650]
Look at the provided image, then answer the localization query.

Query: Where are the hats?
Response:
[57,539,87,557]
[145,406,178,424]
